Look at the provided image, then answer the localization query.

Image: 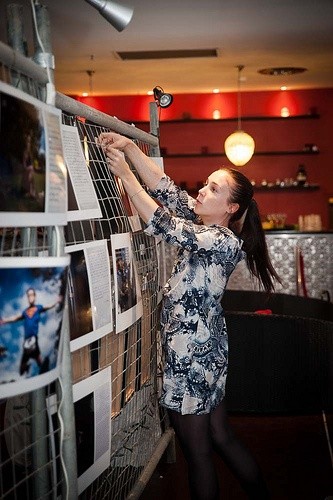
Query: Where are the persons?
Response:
[0,287,62,375]
[100,132,284,500]
[115,256,132,312]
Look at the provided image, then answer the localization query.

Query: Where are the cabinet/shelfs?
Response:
[126,115,321,191]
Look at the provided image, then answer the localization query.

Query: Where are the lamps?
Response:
[84,0,134,32]
[224,64,256,166]
[153,85,173,109]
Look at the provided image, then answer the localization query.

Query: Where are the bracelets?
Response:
[129,188,146,200]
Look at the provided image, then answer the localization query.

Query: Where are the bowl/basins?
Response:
[266,214,288,228]
